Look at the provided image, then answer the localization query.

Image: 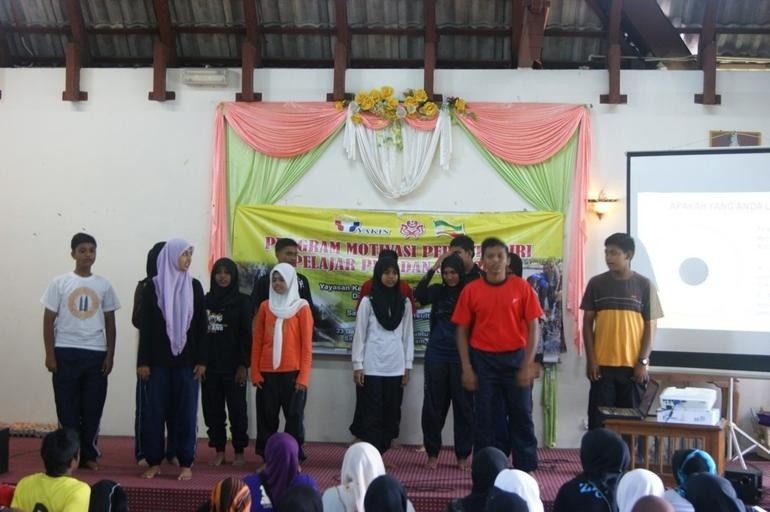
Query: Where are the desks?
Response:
[601,413,727,489]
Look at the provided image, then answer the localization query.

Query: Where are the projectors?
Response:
[659,386,716,410]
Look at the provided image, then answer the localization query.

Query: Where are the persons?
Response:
[413,234,544,475]
[553,428,767,512]
[347,248,416,469]
[454,446,543,512]
[131,237,314,481]
[10,427,126,511]
[577,233,664,434]
[206,432,413,512]
[39,232,122,469]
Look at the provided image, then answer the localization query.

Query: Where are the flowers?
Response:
[333,87,480,152]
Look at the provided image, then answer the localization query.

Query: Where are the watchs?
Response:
[638,357,649,365]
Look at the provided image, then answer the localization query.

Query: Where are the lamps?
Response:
[585,181,620,222]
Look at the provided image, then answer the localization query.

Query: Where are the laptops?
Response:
[597,379,659,420]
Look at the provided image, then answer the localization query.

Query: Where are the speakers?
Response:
[0,427,10,474]
[725,467,763,504]
